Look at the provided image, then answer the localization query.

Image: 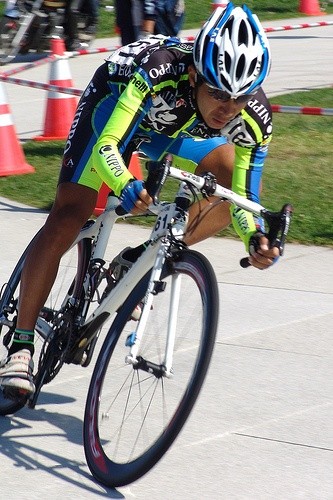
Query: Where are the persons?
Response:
[0,6,284,394]
[6,0,184,52]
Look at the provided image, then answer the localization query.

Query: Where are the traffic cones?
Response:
[211,0,229,11]
[296,0,328,16]
[31,37,78,141]
[0,78,35,178]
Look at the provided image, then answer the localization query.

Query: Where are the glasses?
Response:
[207,88,254,104]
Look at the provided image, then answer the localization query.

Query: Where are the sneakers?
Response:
[111,247,142,322]
[0,349,35,399]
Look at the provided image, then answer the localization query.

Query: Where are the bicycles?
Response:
[0,149,292,488]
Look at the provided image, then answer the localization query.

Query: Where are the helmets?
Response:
[193,2,272,100]
